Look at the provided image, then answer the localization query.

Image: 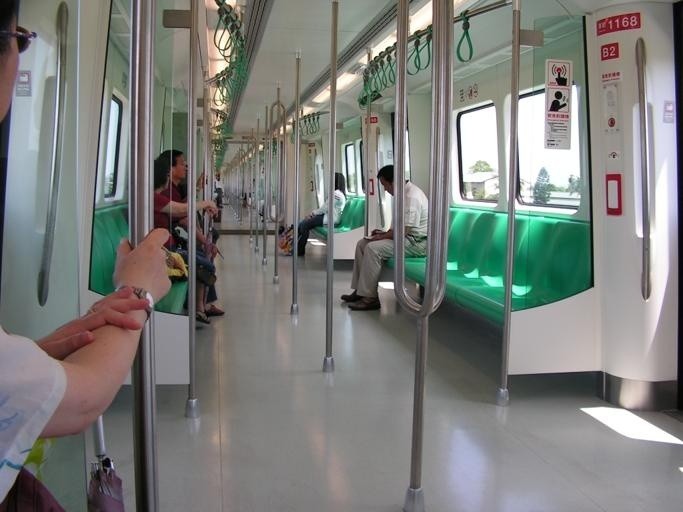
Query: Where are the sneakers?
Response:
[341,289,363,302]
[349,297,380,310]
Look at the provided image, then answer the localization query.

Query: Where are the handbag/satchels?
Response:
[279,224,305,256]
[174,247,210,270]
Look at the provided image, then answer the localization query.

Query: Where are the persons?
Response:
[1,1,173,510]
[154,148,226,324]
[295,172,346,256]
[340,165,429,311]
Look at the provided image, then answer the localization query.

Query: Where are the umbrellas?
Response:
[86,414,126,511]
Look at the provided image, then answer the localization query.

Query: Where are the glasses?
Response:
[1,25,37,53]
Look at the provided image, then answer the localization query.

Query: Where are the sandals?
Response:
[195,311,211,323]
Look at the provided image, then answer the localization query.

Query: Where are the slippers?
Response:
[204,303,225,316]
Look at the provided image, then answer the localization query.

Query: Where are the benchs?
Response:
[311,190,365,241]
[87,201,196,316]
[379,205,591,333]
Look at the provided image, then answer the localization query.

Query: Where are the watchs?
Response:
[116,284,155,317]
